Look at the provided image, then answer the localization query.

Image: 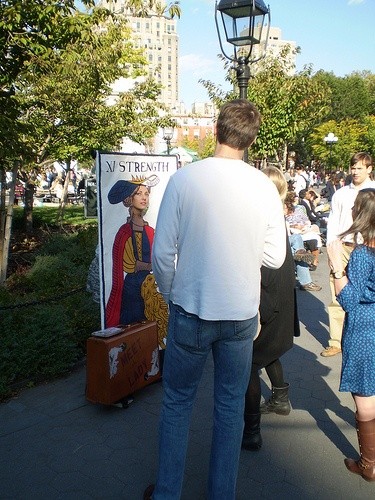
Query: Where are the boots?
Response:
[242,412,262,448]
[345,418,375,482]
[260,382,290,415]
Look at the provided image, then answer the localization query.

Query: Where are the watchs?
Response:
[332,271,346,279]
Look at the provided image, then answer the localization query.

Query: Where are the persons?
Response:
[241,167,300,451]
[320,152,374,357]
[144,99,286,500]
[14,164,375,291]
[327,188,375,482]
[106,175,160,327]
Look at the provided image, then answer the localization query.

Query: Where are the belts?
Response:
[345,242,356,247]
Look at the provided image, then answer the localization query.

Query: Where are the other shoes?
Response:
[321,346,342,356]
[144,485,153,500]
[300,282,321,291]
